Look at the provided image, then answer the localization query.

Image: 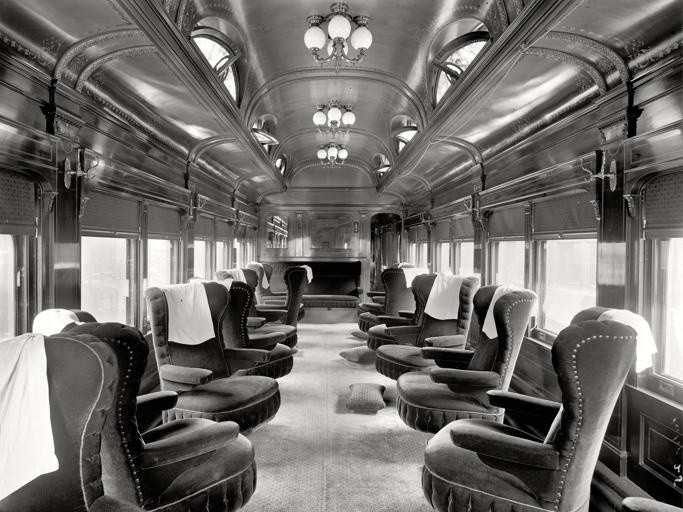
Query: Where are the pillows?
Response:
[350,330,367,340]
[338,345,376,363]
[345,383,385,412]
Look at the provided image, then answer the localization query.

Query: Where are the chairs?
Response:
[357,267,429,333]
[188,276,298,379]
[0,333,148,512]
[420,306,657,512]
[622,497,682,512]
[366,272,453,352]
[144,283,280,433]
[254,265,313,330]
[244,260,305,322]
[394,285,538,448]
[213,268,297,350]
[30,308,256,512]
[269,261,364,308]
[356,262,414,318]
[374,274,481,381]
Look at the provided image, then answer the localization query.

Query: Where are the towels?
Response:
[163,281,217,347]
[299,264,313,285]
[0,332,60,500]
[596,309,656,373]
[481,286,519,340]
[215,268,247,292]
[402,268,430,288]
[247,261,270,290]
[424,273,464,320]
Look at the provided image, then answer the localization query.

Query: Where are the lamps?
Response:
[312,99,355,135]
[316,142,348,168]
[303,1,372,72]
